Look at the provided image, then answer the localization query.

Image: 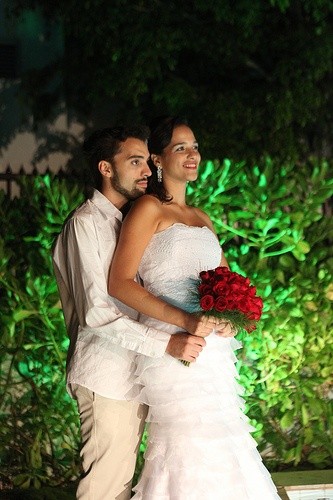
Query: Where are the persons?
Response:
[108,123,280,500]
[51,126,207,500]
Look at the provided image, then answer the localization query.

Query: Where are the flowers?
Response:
[177,265,263,367]
